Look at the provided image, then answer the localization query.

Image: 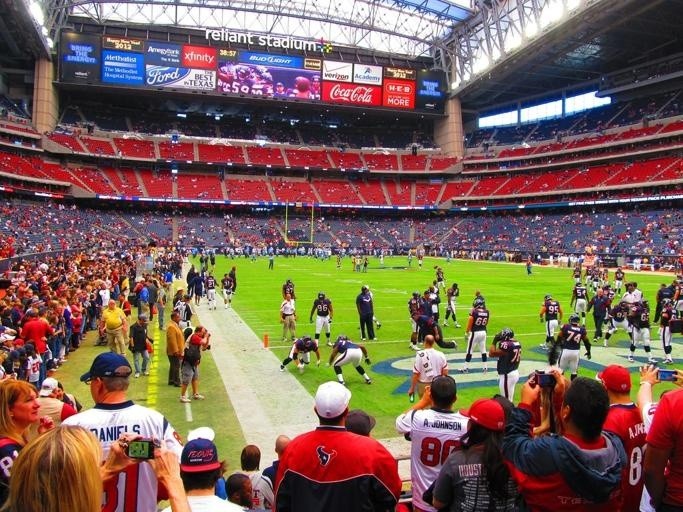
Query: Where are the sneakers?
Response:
[143,370,149,377]
[408,322,462,351]
[647,356,658,364]
[628,353,634,362]
[481,366,487,375]
[456,367,468,374]
[180,395,191,402]
[278,336,377,385]
[662,358,673,364]
[133,372,140,378]
[539,343,547,349]
[592,335,639,348]
[192,393,203,400]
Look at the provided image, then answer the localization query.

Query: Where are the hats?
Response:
[598,364,631,393]
[345,408,376,434]
[79,351,132,382]
[458,398,505,432]
[314,380,351,419]
[0,333,34,360]
[138,313,149,322]
[38,376,58,397]
[178,437,221,473]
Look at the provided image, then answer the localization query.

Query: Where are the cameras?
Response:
[657,369,677,382]
[205,344,210,350]
[127,440,154,460]
[203,332,211,338]
[535,373,556,387]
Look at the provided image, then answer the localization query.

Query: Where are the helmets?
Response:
[544,295,552,300]
[317,292,325,300]
[472,298,485,308]
[568,313,580,324]
[501,328,514,339]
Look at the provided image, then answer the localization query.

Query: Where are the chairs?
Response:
[0,91,681,270]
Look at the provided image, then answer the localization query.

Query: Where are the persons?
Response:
[217,62,321,99]
[0,99,683,511]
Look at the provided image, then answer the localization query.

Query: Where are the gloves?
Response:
[492,333,505,345]
[584,349,592,359]
[327,317,332,323]
